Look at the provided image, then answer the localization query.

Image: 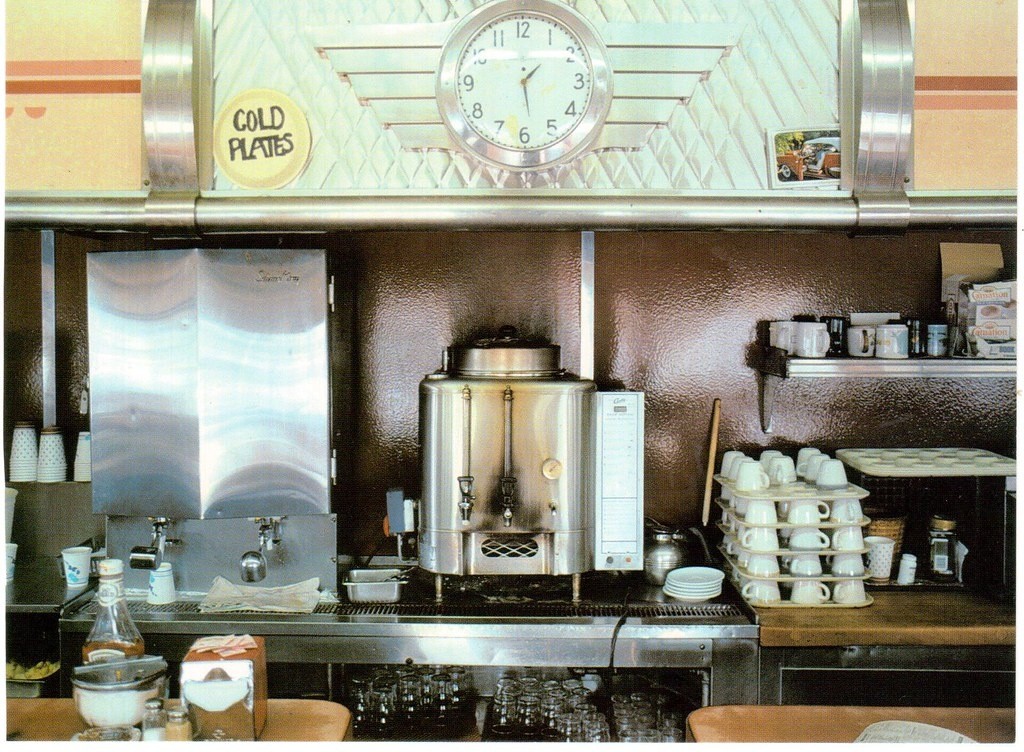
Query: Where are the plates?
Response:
[662,566,725,602]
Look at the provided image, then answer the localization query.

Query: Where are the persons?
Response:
[803,143,823,171]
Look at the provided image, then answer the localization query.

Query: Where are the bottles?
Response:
[141,698,167,740]
[897,554,917,585]
[165,711,192,740]
[643,516,688,587]
[82,559,146,664]
[926,515,959,583]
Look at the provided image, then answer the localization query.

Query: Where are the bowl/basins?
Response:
[70,654,169,727]
[71,724,141,741]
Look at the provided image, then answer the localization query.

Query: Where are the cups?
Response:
[719,447,867,605]
[757,311,948,359]
[864,536,895,581]
[5,420,91,589]
[146,562,176,605]
[348,664,683,743]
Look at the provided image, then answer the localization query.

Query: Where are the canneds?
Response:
[927,517,958,584]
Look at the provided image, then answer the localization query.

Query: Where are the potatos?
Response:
[6,656,61,681]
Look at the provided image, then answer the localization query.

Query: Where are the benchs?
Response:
[808,146,836,170]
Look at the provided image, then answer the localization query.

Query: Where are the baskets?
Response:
[863,507,905,563]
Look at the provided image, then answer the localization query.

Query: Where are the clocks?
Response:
[436,0,614,172]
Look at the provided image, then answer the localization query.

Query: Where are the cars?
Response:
[776,137,841,179]
